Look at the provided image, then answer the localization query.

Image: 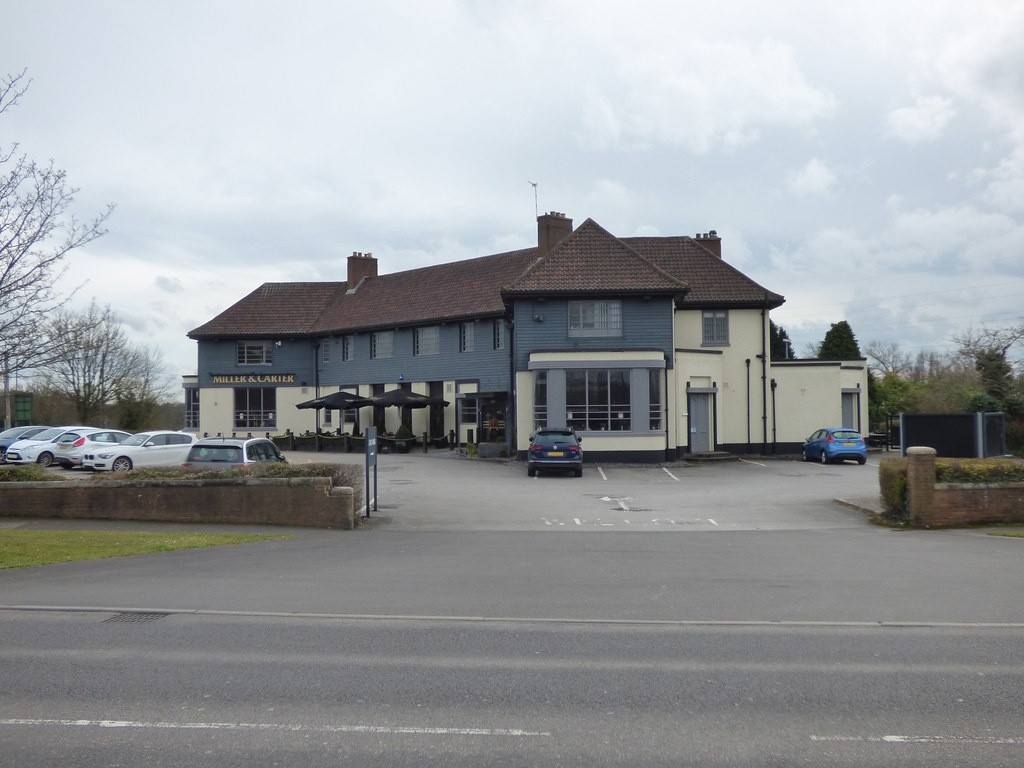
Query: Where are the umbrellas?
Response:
[296,389,451,435]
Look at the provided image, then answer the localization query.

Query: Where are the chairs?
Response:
[538,434,552,444]
[560,433,573,442]
[213,448,231,460]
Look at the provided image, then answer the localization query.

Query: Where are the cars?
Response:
[55,428,134,470]
[81,430,199,474]
[0,426,54,467]
[179,436,289,473]
[801,428,867,465]
[5,425,101,468]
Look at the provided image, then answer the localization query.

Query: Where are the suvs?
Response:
[527,426,583,477]
